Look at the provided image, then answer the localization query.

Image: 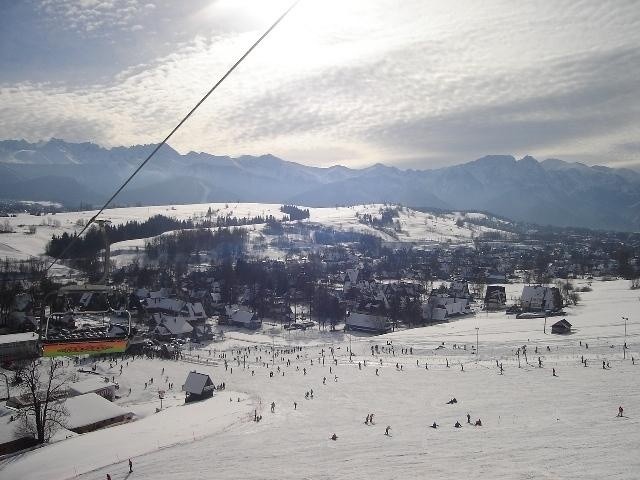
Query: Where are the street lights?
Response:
[622,316,629,336]
[475,327,481,354]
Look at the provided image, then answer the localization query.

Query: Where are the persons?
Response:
[128,459,134,473]
[106,473,112,480]
[88,339,635,440]
